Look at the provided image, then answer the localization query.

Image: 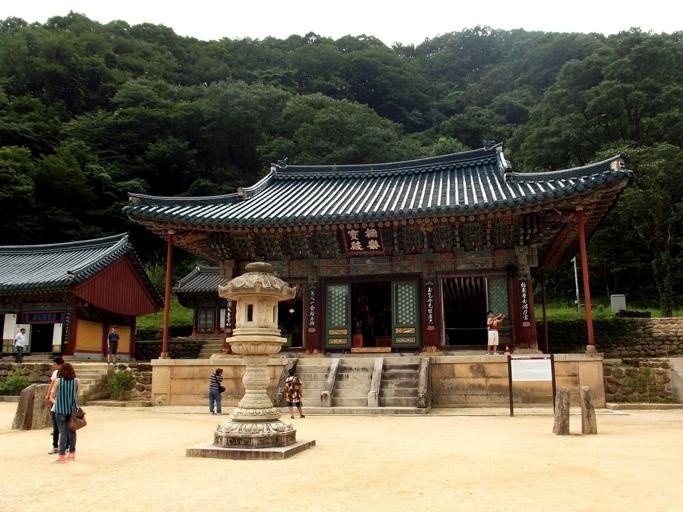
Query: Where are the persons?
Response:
[283,369,306,418]
[486,310,505,355]
[45,357,65,454]
[52,363,79,461]
[107,326,119,366]
[208,368,223,416]
[13,329,28,363]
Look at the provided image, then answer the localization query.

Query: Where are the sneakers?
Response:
[51,452,76,462]
[291,415,305,419]
[49,446,58,455]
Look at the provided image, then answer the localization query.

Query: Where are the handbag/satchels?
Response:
[67,405,87,431]
[219,386,226,394]
